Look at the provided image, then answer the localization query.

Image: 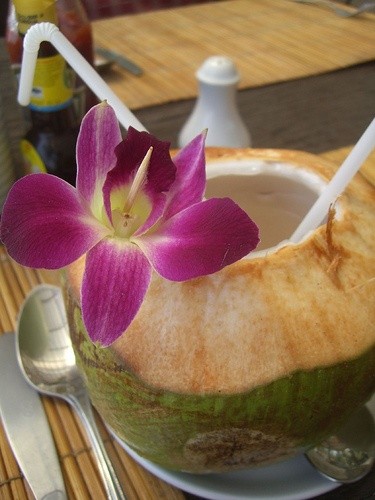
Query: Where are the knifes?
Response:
[0,331,70,499]
[93,48,142,78]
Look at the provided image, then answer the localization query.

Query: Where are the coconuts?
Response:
[70,146,375,475]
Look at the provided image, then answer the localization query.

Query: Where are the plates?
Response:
[99,394,375,500]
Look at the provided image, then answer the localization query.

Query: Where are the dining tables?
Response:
[0,0,375,500]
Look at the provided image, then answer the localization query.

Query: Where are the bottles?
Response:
[175,55,252,155]
[6,1,94,135]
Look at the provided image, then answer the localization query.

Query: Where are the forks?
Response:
[301,1,371,17]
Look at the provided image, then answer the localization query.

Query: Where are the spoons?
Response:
[16,284,133,500]
[89,55,112,73]
[304,405,375,482]
